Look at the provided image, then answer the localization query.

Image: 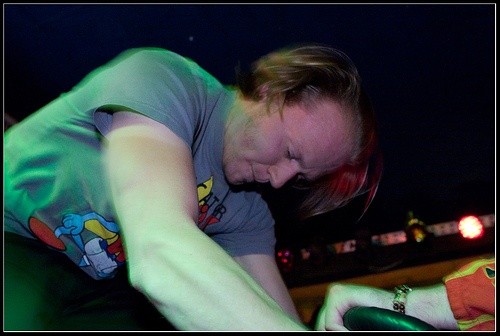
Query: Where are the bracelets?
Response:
[392,285,411,315]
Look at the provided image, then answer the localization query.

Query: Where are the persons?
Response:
[318,255,496,333]
[3,46,363,331]
